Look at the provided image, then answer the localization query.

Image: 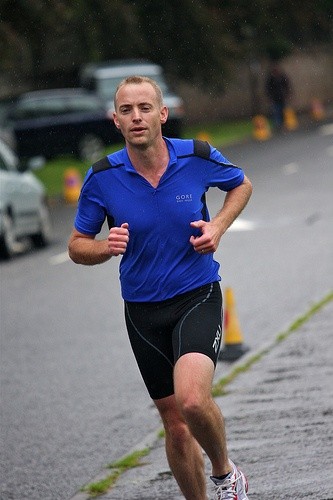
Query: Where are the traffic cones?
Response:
[254,115,271,141]
[62,168,82,201]
[283,106,297,131]
[216,285,250,361]
[311,97,324,122]
[198,133,211,146]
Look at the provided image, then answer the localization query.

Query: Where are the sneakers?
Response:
[210,457,248,500]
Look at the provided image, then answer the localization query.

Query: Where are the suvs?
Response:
[89,65,187,144]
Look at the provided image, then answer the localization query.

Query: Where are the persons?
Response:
[67,73,257,500]
[263,55,296,141]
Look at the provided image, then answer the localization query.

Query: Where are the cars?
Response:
[0,141,52,260]
[7,88,127,163]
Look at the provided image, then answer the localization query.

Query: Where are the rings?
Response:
[201,249,205,254]
